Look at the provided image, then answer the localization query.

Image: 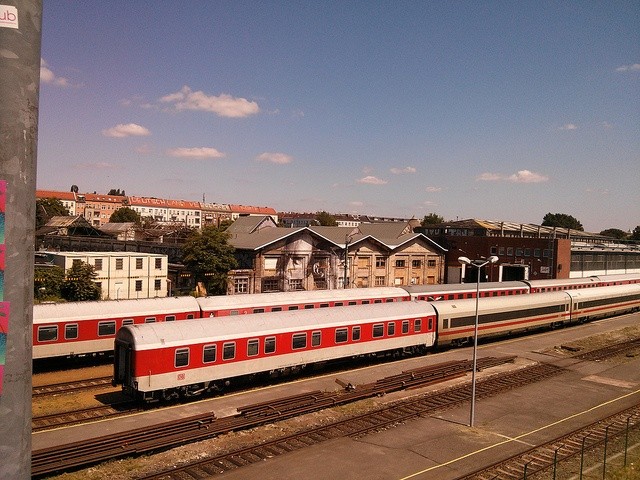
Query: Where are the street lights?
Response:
[457,256,499,427]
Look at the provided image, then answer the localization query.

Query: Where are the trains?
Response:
[32,273,640,360]
[111,283,640,405]
